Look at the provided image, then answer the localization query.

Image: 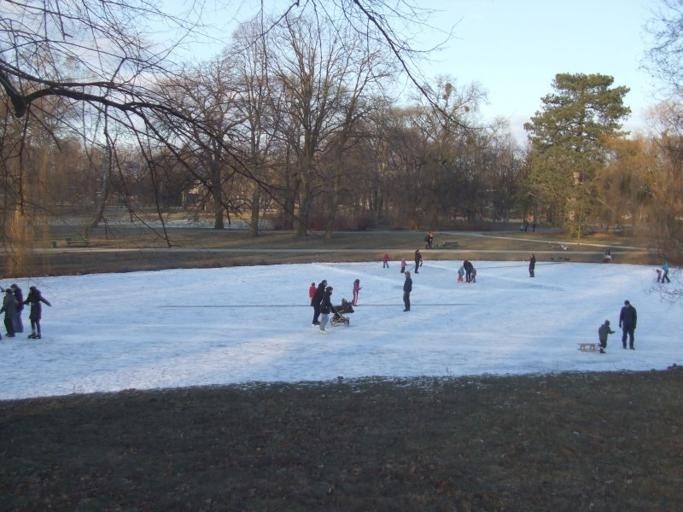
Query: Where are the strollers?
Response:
[329,306,349,328]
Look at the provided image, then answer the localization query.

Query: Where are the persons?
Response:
[22,286,52,339]
[528,254,535,278]
[655,268,661,281]
[618,300,637,350]
[305,231,478,340]
[9,283,23,333]
[0,288,19,337]
[661,263,671,284]
[598,320,614,354]
